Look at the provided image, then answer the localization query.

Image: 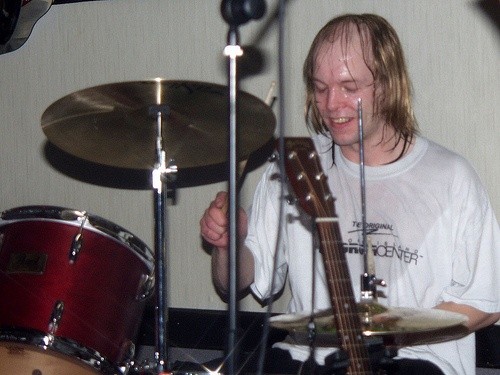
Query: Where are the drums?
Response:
[1,205,157,375]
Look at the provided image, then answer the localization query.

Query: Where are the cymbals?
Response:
[265,296,468,347]
[40,81,277,169]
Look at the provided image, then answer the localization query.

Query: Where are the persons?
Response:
[200,13,500,375]
